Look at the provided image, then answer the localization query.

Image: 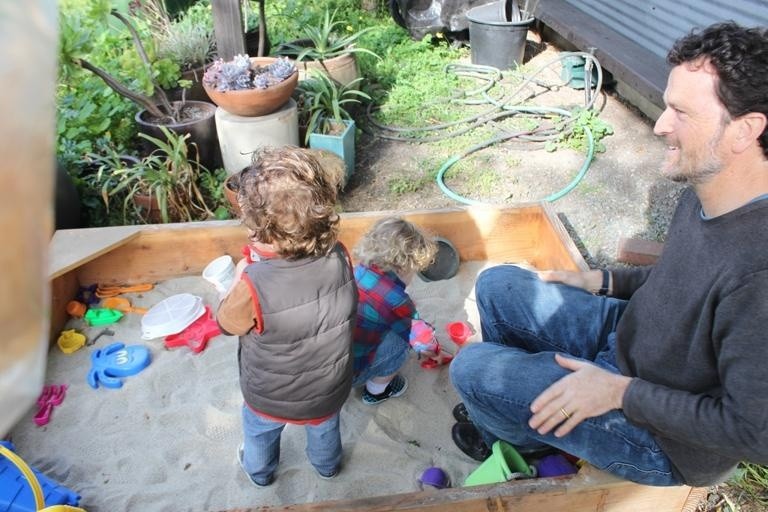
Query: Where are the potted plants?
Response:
[135,1,209,100]
[116,127,199,225]
[66,10,214,177]
[276,5,363,117]
[241,3,271,61]
[201,53,299,117]
[223,172,244,219]
[298,76,362,192]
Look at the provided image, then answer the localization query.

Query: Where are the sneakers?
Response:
[361,374,408,405]
[237,443,268,487]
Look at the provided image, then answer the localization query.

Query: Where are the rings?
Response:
[560,407,569,419]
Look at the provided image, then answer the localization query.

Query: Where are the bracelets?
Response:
[595,267,610,296]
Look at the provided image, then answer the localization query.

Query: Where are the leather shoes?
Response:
[453,403,555,461]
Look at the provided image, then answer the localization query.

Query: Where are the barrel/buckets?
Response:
[459,440,537,489]
[466,6,536,71]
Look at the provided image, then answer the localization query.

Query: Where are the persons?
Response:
[449,20,766,488]
[350,215,447,405]
[212,143,360,489]
